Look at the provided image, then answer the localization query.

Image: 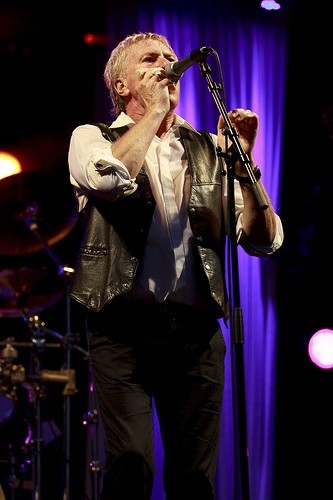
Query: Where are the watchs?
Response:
[234,166,262,183]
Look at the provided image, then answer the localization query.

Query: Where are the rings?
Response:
[152,71,162,78]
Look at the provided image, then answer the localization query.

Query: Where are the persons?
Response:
[68,31,284,500]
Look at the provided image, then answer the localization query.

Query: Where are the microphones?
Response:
[165,47,210,82]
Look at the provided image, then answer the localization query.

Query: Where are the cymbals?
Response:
[0,266,66,318]
[0,166,81,255]
